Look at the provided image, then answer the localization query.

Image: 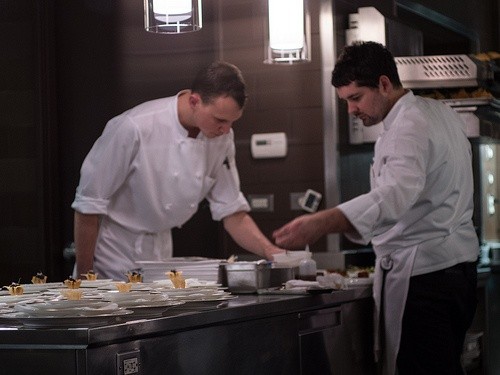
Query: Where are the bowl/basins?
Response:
[225,263,269,294]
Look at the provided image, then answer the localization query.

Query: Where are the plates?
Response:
[2,277,228,327]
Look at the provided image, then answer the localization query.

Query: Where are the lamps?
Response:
[143,0,203,35]
[264,0,311,64]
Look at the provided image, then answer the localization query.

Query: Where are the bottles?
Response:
[300,252,317,284]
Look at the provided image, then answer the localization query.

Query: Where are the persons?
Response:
[71,62,287,281]
[272,40,480,375]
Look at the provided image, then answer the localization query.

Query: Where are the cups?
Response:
[270,267,300,288]
